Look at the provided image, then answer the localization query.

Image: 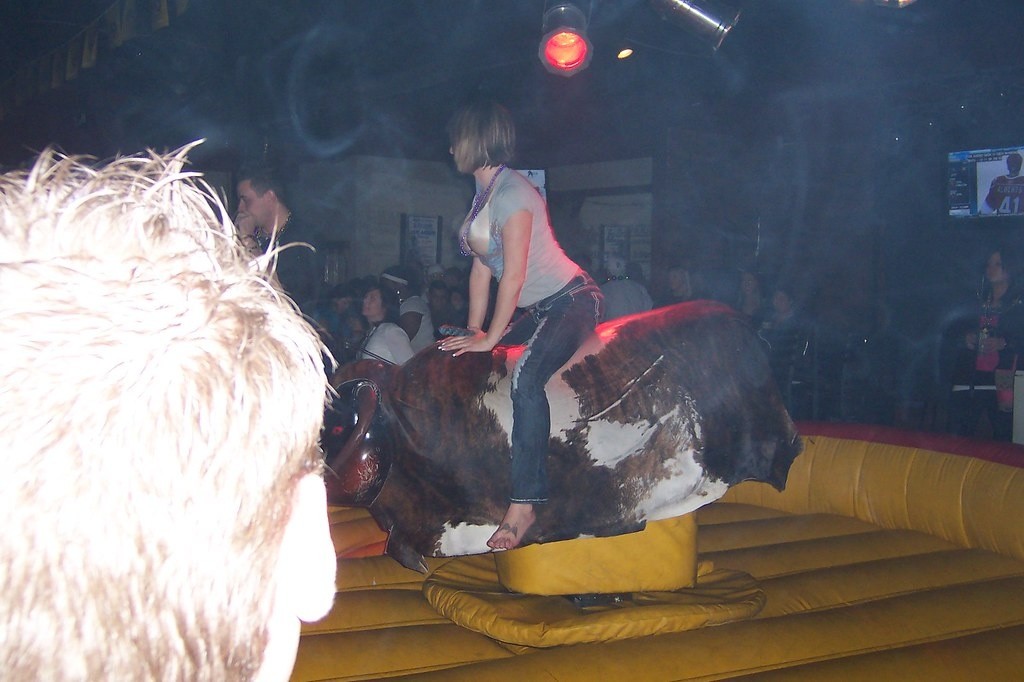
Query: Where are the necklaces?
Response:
[982,283,1009,338]
[256,210,292,243]
[459,164,507,258]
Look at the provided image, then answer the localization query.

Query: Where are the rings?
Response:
[980,345,984,348]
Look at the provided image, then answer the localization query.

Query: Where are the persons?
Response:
[232,159,319,323]
[318,266,500,338]
[434,98,603,549]
[379,265,435,356]
[947,246,1024,441]
[562,238,982,436]
[354,285,415,365]
[977,152,1024,215]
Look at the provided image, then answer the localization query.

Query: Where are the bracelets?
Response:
[999,336,1008,352]
[238,234,259,253]
